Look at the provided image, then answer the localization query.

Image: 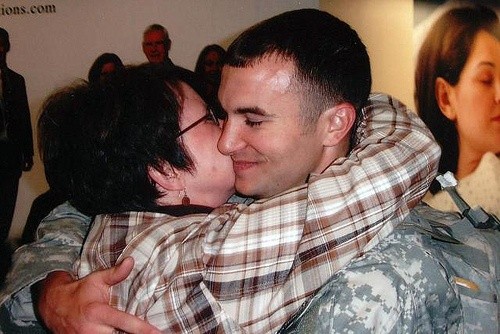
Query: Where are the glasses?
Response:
[173,107,220,141]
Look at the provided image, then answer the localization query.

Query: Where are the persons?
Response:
[414,5,499,219]
[0,9,464,334]
